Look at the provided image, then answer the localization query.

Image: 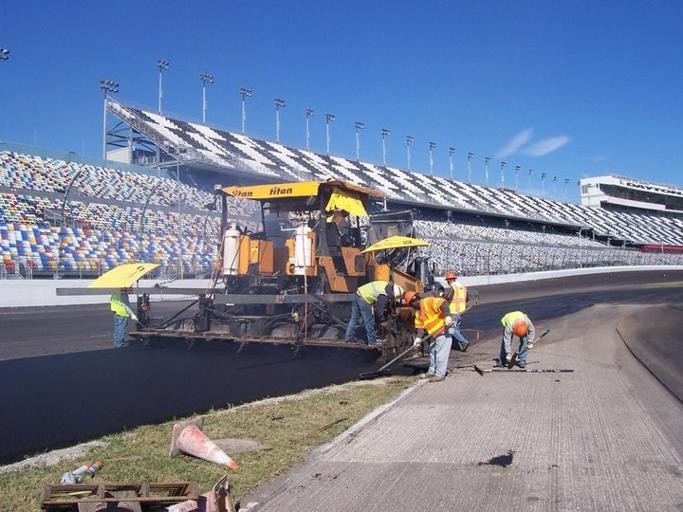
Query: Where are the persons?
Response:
[110,287,139,349]
[372,251,391,281]
[345,281,404,347]
[404,291,453,382]
[442,272,469,352]
[500,311,535,368]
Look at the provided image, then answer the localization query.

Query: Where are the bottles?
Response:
[62,460,101,482]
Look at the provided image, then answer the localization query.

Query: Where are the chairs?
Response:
[271,140,409,201]
[104,102,270,278]
[410,171,683,278]
[0,150,104,274]
[0,101,683,279]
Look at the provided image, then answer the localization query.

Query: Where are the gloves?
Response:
[413,337,421,350]
[506,355,512,363]
[526,342,533,351]
[445,316,453,327]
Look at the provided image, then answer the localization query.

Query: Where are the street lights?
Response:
[404,134,415,170]
[463,149,580,202]
[154,58,170,112]
[272,95,286,144]
[428,140,436,174]
[380,127,391,165]
[97,76,121,160]
[304,107,315,149]
[352,120,365,161]
[0,47,10,63]
[447,145,456,178]
[198,71,215,123]
[238,85,254,136]
[323,111,335,154]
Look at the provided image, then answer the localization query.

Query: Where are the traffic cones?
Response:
[165,414,245,474]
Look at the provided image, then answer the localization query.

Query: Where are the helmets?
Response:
[513,322,528,337]
[445,272,458,281]
[404,290,418,305]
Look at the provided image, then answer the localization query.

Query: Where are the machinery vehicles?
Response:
[130,176,440,372]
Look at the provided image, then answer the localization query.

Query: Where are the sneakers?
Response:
[461,341,469,352]
[423,373,446,382]
[518,362,526,369]
[497,361,506,368]
